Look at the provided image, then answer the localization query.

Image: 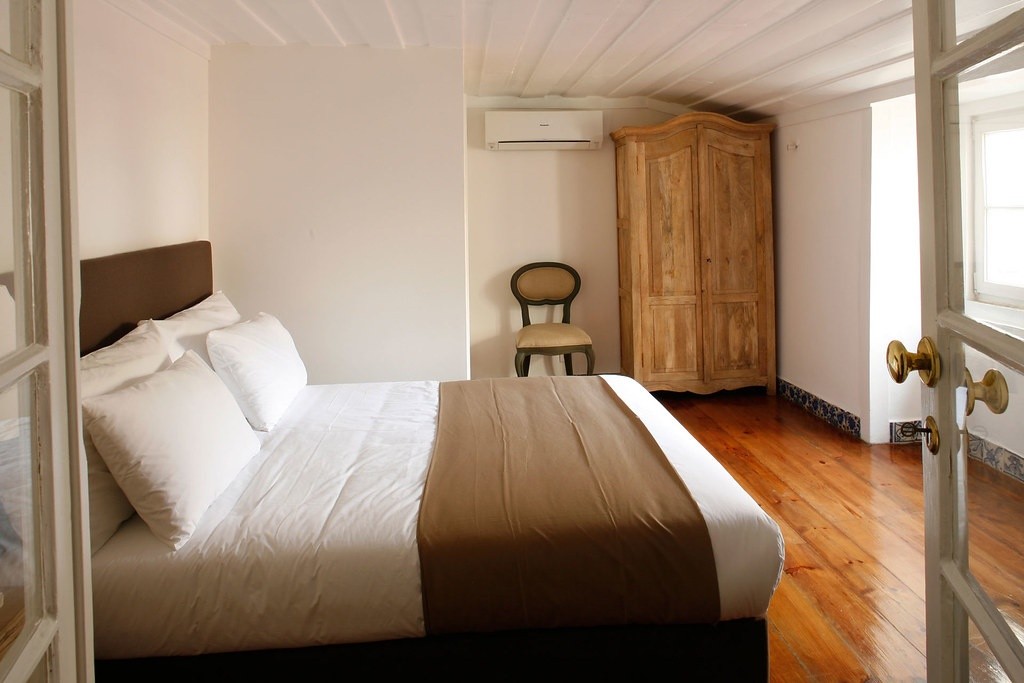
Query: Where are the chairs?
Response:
[509,263,597,375]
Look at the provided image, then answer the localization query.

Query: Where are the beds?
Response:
[0,239,788,682]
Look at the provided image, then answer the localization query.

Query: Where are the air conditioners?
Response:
[483,108,604,151]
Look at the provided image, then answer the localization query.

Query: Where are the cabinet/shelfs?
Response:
[610,111,781,401]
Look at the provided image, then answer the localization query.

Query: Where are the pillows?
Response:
[80,347,262,550]
[205,313,307,432]
[137,288,243,362]
[77,319,192,558]
